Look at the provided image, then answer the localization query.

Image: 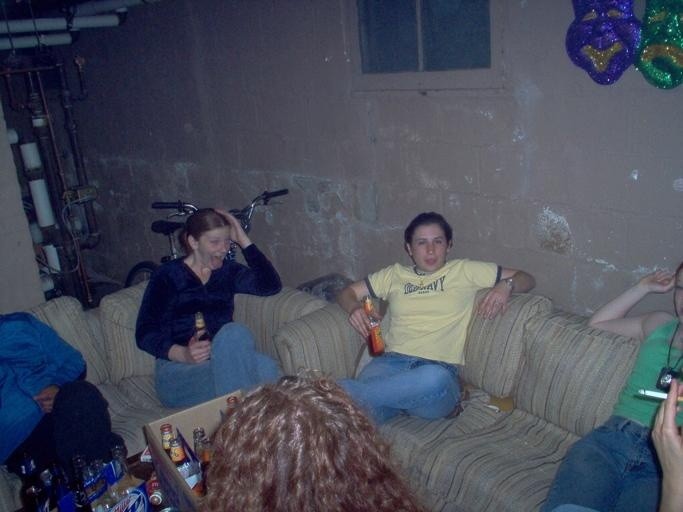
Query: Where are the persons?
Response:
[136,208,279,409]
[197,373,422,512]
[539,263,683,512]
[651,378,683,512]
[0,312,128,485]
[335,212,535,425]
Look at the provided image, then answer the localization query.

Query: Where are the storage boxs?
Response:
[144,389,240,512]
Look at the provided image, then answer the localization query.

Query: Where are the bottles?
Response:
[362,294,386,356]
[160,423,176,458]
[18,438,137,512]
[193,395,241,469]
[185,460,206,496]
[192,310,212,362]
[167,435,190,478]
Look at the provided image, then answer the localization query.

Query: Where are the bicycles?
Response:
[121,187,290,291]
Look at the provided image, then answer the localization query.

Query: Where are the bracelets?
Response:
[503,278,514,290]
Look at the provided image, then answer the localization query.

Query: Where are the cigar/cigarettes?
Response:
[638,389,683,402]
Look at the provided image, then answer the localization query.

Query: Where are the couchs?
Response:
[273,299,639,511]
[0,287,329,511]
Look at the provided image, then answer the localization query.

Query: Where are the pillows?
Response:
[514,310,640,438]
[460,288,553,399]
[29,296,108,385]
[99,279,149,385]
